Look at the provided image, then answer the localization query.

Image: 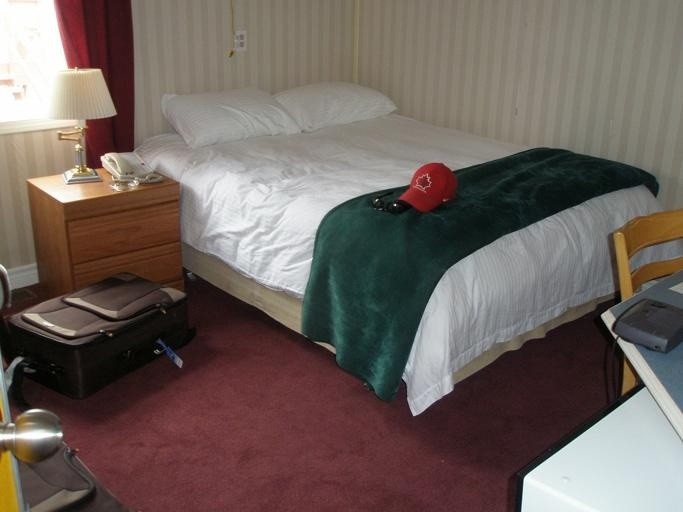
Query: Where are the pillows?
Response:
[163,81,396,150]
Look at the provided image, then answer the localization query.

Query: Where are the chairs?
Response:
[610,211,682,390]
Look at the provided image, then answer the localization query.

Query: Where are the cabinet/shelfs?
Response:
[29,168,185,312]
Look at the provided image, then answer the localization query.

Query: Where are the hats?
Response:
[397,162,458,213]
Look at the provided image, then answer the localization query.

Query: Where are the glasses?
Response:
[371,192,411,214]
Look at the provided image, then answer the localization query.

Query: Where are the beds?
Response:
[139,116,664,398]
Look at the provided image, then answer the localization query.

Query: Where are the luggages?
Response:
[15,439,132,511]
[6,271,197,399]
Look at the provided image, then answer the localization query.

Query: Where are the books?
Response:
[601,269,683,441]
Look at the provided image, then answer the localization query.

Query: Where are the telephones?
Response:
[99,152,152,179]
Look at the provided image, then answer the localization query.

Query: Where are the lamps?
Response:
[46,65,115,187]
